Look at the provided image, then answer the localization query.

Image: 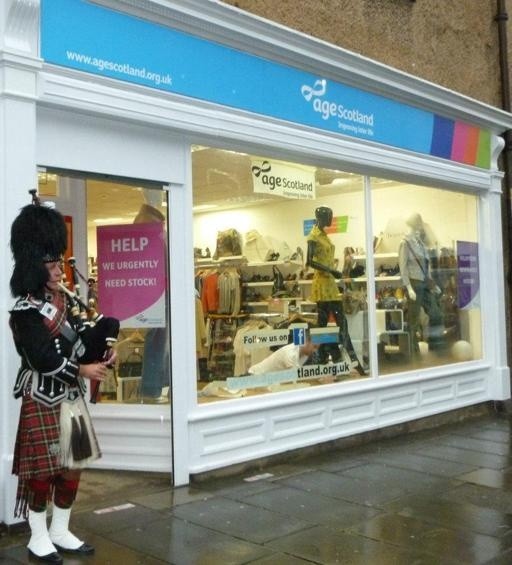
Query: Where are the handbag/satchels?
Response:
[423,276,437,290]
[56,395,103,470]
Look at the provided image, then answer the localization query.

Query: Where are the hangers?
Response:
[113,330,147,347]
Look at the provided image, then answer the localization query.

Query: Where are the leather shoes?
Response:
[243,270,314,281]
[375,262,400,276]
[28,541,96,565]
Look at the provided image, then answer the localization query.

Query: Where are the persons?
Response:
[132,204,170,403]
[307,206,368,377]
[8,251,117,565]
[242,332,336,385]
[398,213,449,364]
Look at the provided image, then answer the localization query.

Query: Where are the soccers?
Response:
[450,340,473,362]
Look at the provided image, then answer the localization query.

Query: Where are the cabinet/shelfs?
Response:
[203,248,477,373]
